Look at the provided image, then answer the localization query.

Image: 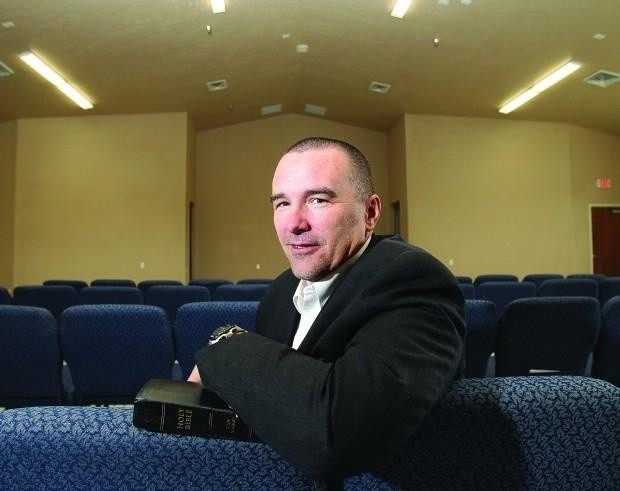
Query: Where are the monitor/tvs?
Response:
[133,377,267,444]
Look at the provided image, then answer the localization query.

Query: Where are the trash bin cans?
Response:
[208,323,246,344]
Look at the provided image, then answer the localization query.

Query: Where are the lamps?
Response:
[14,44,96,114]
[496,55,585,117]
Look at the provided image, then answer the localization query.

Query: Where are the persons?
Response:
[184,136,470,480]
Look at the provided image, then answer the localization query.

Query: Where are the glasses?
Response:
[14,44,96,114]
[496,55,585,117]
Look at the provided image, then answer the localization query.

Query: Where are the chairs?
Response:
[0,274,619,490]
